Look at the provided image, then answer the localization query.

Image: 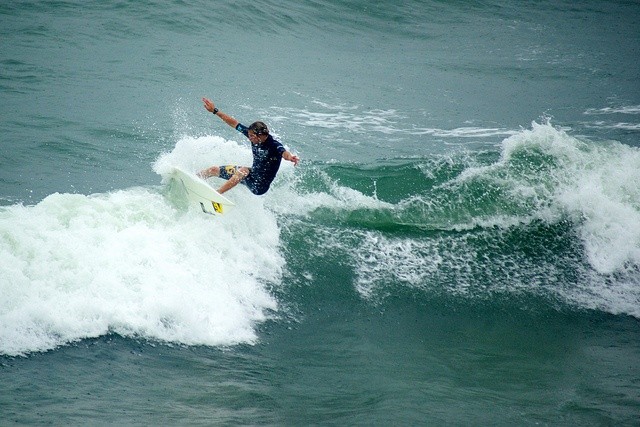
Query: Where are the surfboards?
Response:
[163,167,235,217]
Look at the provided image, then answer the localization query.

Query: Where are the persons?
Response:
[197,96,300,194]
